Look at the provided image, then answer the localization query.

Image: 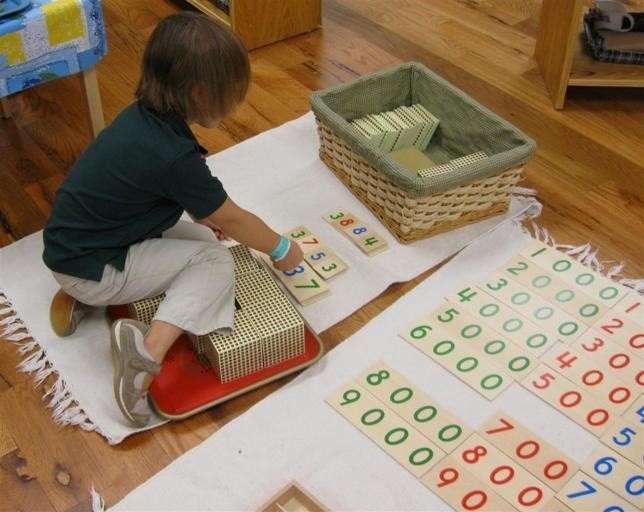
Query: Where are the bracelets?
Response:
[269,237,291,263]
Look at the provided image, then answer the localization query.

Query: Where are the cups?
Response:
[591,1,635,33]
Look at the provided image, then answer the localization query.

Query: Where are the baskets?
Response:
[309,61,536,245]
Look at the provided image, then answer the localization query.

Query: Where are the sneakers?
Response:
[110,318,162,427]
[50,288,97,336]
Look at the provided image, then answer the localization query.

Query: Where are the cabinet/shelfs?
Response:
[534,0,644,109]
[185,0,323,53]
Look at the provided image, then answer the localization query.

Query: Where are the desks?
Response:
[0,0,109,139]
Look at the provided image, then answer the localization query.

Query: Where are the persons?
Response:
[42,12,303,426]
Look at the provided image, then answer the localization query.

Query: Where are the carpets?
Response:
[89,222,644,512]
[0,111,542,445]
